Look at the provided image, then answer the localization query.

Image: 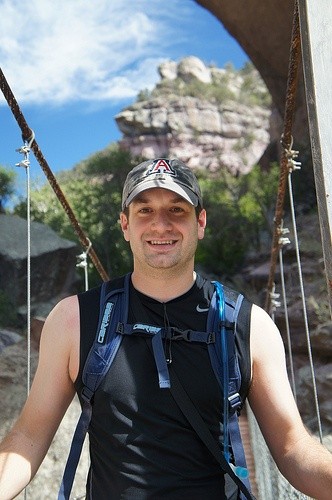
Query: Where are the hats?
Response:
[122,158,203,211]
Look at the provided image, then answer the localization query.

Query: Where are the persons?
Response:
[0,156,332,500]
